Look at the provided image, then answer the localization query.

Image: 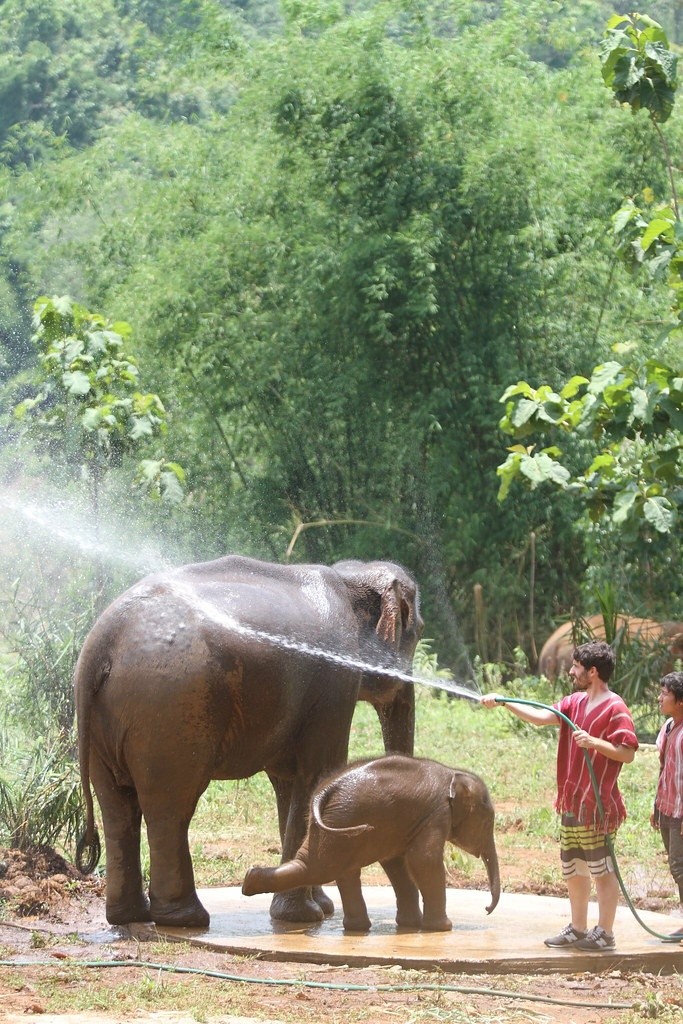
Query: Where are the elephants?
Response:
[74,553,426,926]
[539,613,682,695]
[243,757,502,932]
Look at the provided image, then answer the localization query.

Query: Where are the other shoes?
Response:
[661,930,683,942]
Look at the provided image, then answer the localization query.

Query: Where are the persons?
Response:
[481,641,639,952]
[650,672,683,946]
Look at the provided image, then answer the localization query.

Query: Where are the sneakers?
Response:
[575,926,617,951]
[544,923,588,948]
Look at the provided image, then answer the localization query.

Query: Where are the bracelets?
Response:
[501,702,505,706]
